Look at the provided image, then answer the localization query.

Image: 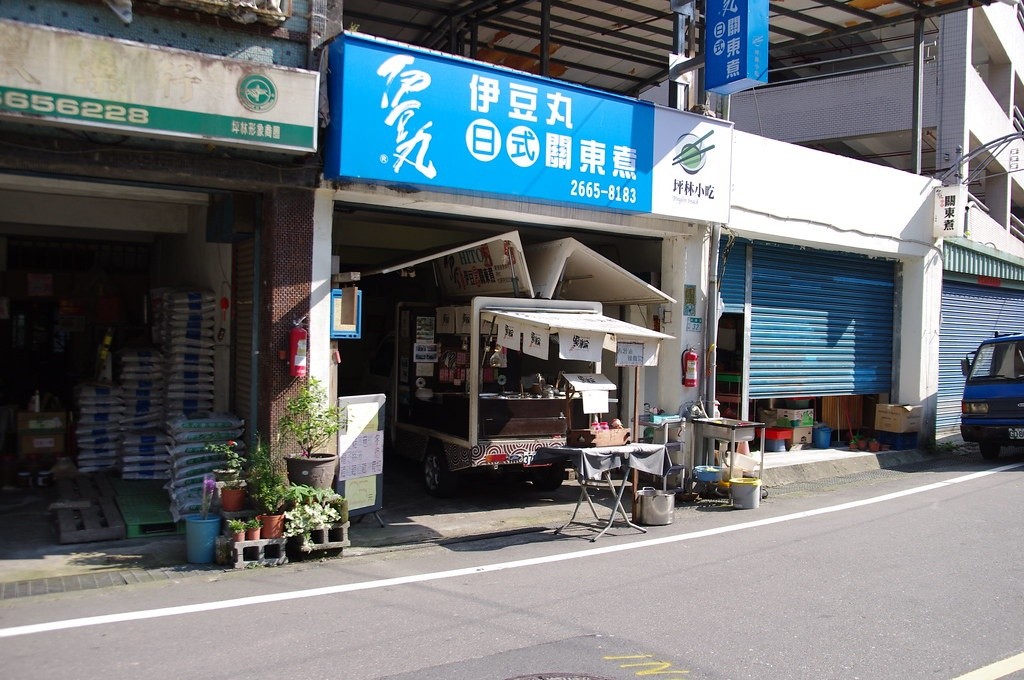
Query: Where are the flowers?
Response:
[200,473,216,519]
[284,482,343,554]
[204,440,247,470]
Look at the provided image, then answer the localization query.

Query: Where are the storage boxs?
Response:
[567,427,632,447]
[874,403,923,449]
[756,408,813,451]
[18,412,66,456]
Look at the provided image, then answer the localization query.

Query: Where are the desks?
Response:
[530,443,673,542]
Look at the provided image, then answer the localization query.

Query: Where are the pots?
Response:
[635,486,676,526]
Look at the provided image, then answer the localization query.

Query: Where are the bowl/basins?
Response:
[695,466,723,482]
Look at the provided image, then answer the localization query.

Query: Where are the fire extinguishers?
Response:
[681,343,700,387]
[278,316,308,378]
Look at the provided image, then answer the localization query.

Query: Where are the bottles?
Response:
[591,422,609,430]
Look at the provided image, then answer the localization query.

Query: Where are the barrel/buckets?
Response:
[729,476,762,510]
[813,426,833,449]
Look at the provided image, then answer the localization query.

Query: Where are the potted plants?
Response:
[221,375,354,541]
[849,433,867,450]
[869,438,880,451]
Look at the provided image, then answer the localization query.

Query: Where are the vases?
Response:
[213,468,240,482]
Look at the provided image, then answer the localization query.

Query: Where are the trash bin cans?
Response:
[812,428,832,449]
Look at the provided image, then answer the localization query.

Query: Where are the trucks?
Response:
[364,305,573,494]
[960,330,1024,460]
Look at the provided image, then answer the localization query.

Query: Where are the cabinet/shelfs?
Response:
[720,372,746,421]
